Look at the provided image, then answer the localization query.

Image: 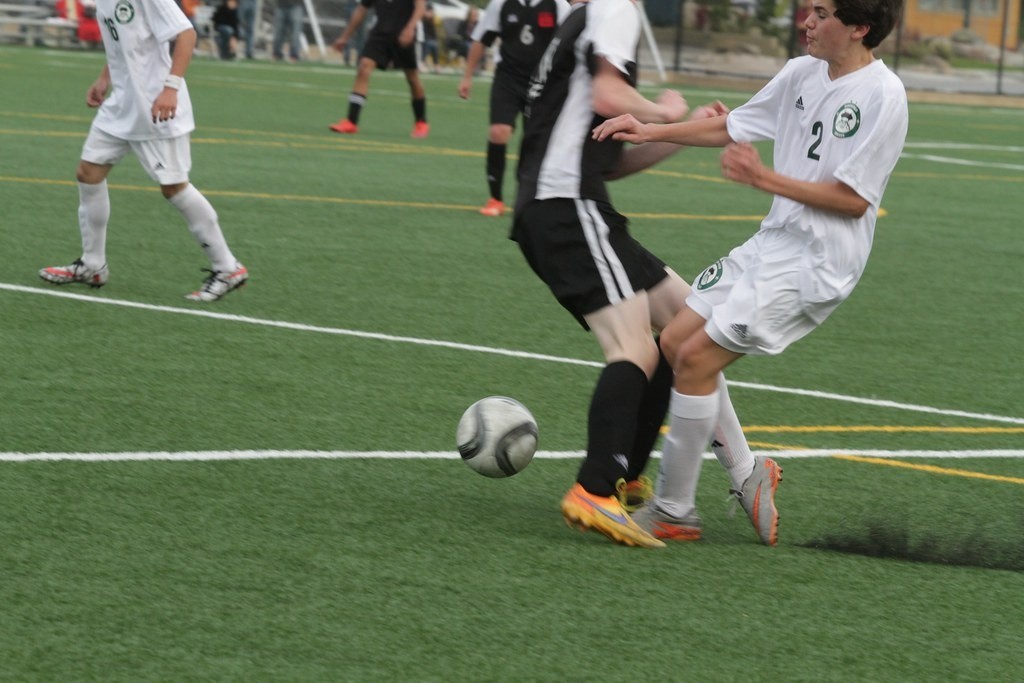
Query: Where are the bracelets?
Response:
[164,74,182,90]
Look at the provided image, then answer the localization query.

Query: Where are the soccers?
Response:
[455,395,539,479]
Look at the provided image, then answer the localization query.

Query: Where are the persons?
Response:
[211,0,244,59]
[420,0,908,550]
[271,0,302,62]
[328,0,430,140]
[38,0,249,303]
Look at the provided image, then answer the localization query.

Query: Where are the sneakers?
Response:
[413,121,429,138]
[615,476,651,513]
[183,260,248,302]
[479,198,505,216]
[330,120,356,132]
[630,501,702,540]
[38,258,110,288]
[562,478,666,548]
[725,456,783,545]
